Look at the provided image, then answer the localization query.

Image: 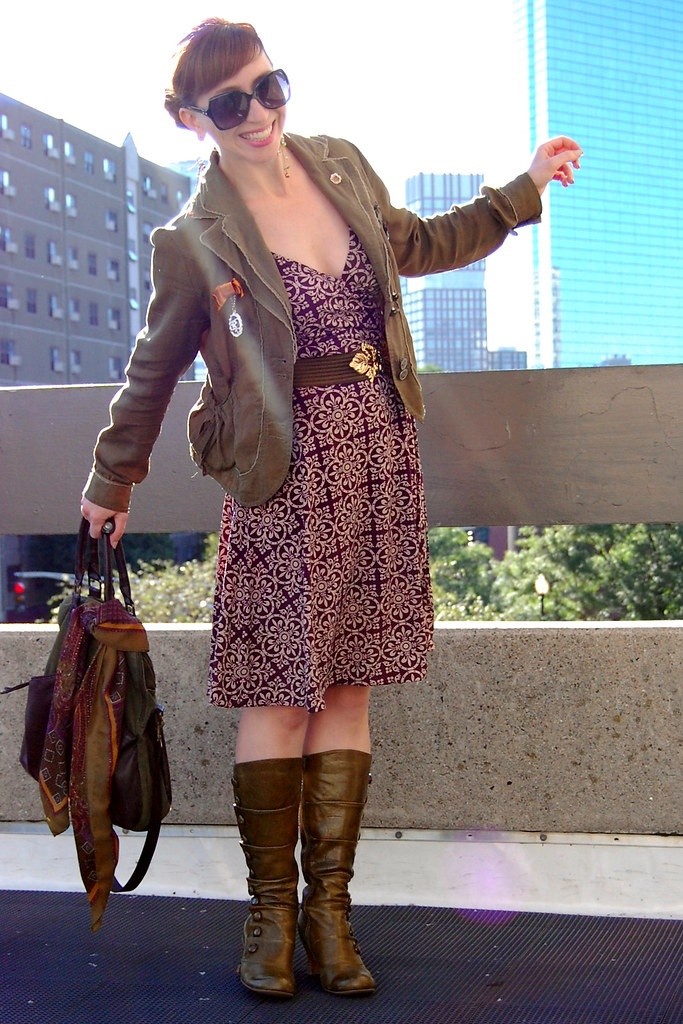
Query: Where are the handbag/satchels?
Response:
[20,516,172,898]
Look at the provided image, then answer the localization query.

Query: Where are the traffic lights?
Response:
[8,576,28,618]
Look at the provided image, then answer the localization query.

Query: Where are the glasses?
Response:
[182,69,291,132]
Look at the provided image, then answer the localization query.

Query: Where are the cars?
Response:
[7,570,102,624]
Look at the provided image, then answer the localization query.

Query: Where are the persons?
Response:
[79,17,583,1000]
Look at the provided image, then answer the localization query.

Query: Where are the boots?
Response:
[295,748,380,993]
[230,756,306,998]
